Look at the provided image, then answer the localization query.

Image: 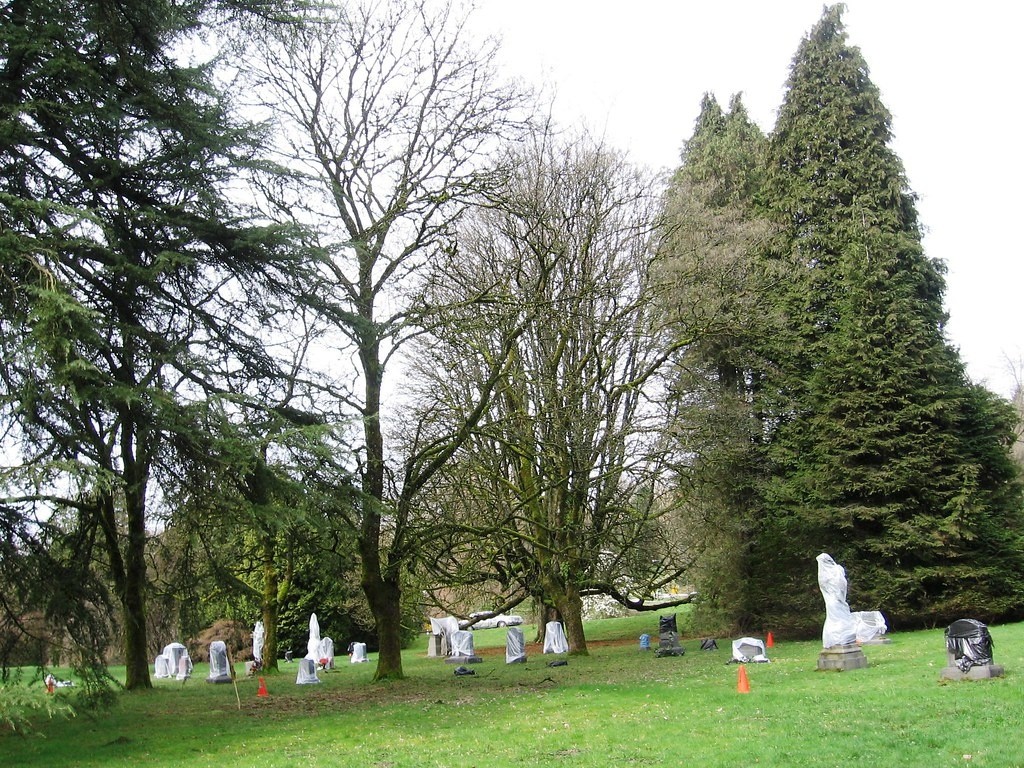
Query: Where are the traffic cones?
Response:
[736,664,751,695]
[44,677,57,696]
[255,677,270,697]
[766,632,777,649]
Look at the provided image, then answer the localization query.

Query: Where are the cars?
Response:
[458,611,523,632]
